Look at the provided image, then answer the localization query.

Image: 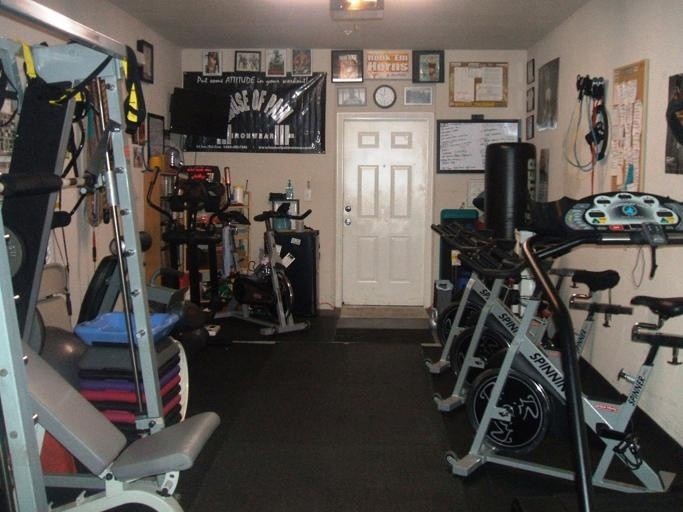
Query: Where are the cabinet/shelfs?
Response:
[143,171,178,285]
[179,191,252,274]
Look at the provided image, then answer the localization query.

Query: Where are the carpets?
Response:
[186,344,469,511]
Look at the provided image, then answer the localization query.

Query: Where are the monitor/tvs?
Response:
[170,88,231,139]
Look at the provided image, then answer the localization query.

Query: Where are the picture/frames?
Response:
[404,87,433,105]
[202,49,223,76]
[265,48,286,77]
[292,47,312,76]
[272,199,299,231]
[611,58,648,193]
[148,113,164,159]
[137,40,153,84]
[331,50,363,82]
[526,115,533,140]
[448,62,508,107]
[526,58,534,84]
[526,87,534,112]
[235,51,261,72]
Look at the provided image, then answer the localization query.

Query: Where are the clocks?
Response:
[373,85,396,108]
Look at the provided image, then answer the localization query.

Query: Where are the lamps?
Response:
[329,1,384,18]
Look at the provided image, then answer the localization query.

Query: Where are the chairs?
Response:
[22,340,220,512]
[36,264,71,331]
